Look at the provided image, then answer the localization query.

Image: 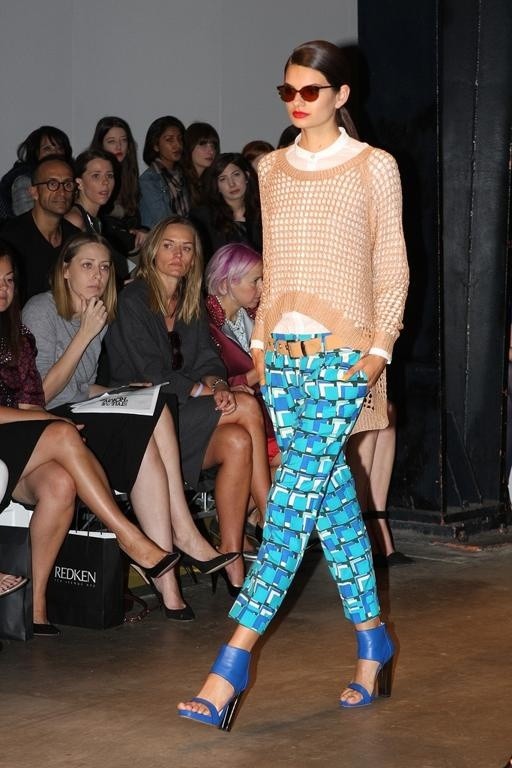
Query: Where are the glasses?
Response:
[36,178,79,191]
[279,84,346,102]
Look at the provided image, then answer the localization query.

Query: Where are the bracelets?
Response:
[194,382,203,400]
[212,378,230,390]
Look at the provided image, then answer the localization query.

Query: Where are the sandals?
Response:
[2,570,28,595]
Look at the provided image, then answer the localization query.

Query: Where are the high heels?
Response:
[121,547,178,582]
[170,544,240,586]
[342,624,394,705]
[209,564,245,600]
[243,520,321,551]
[32,622,61,636]
[178,646,251,732]
[365,510,416,566]
[161,589,196,622]
[209,516,261,561]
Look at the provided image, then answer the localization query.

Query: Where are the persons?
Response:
[1,125,74,216]
[0,243,182,637]
[347,401,415,570]
[242,140,275,173]
[23,231,240,622]
[0,154,85,304]
[95,213,315,596]
[139,115,186,229]
[186,122,220,228]
[179,41,408,731]
[201,244,282,549]
[65,147,153,291]
[276,124,302,149]
[190,153,263,260]
[91,117,142,225]
[0,462,28,596]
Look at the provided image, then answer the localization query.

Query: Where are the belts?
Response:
[271,335,350,357]
